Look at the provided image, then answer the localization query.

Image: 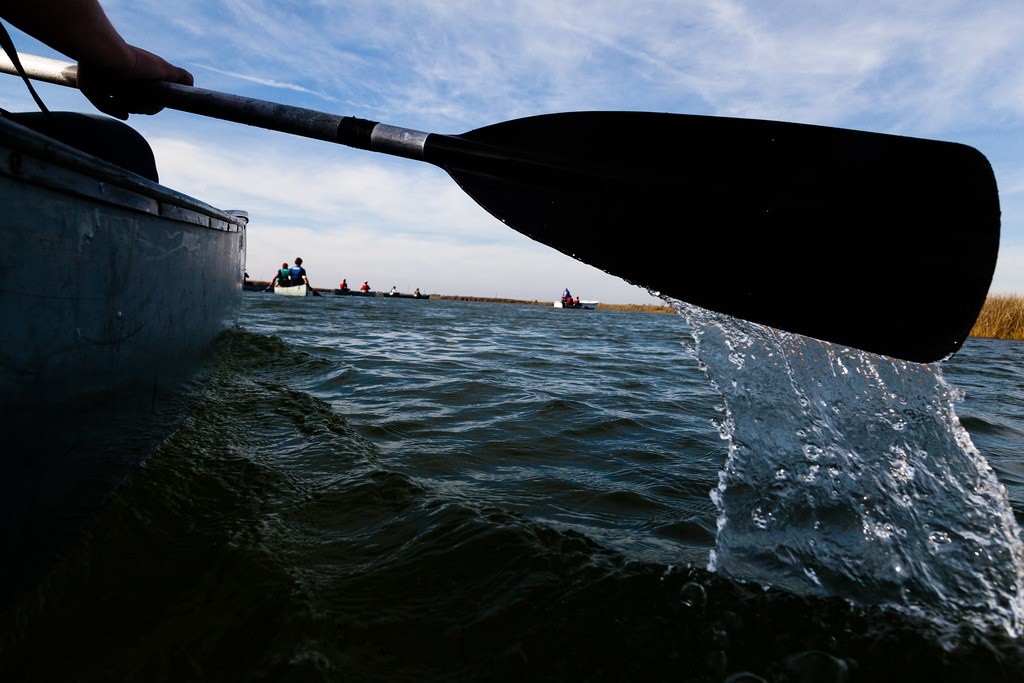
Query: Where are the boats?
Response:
[554,301,600,309]
[383,293,430,299]
[334,289,376,297]
[0,115,248,537]
[274,280,307,296]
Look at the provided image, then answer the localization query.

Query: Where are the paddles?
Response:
[0,49,1003,364]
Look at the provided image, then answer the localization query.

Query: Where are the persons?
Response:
[390,286,396,295]
[0,0,194,183]
[244,273,249,285]
[414,288,421,296]
[562,288,581,305]
[340,279,347,290]
[269,257,308,286]
[360,281,370,292]
